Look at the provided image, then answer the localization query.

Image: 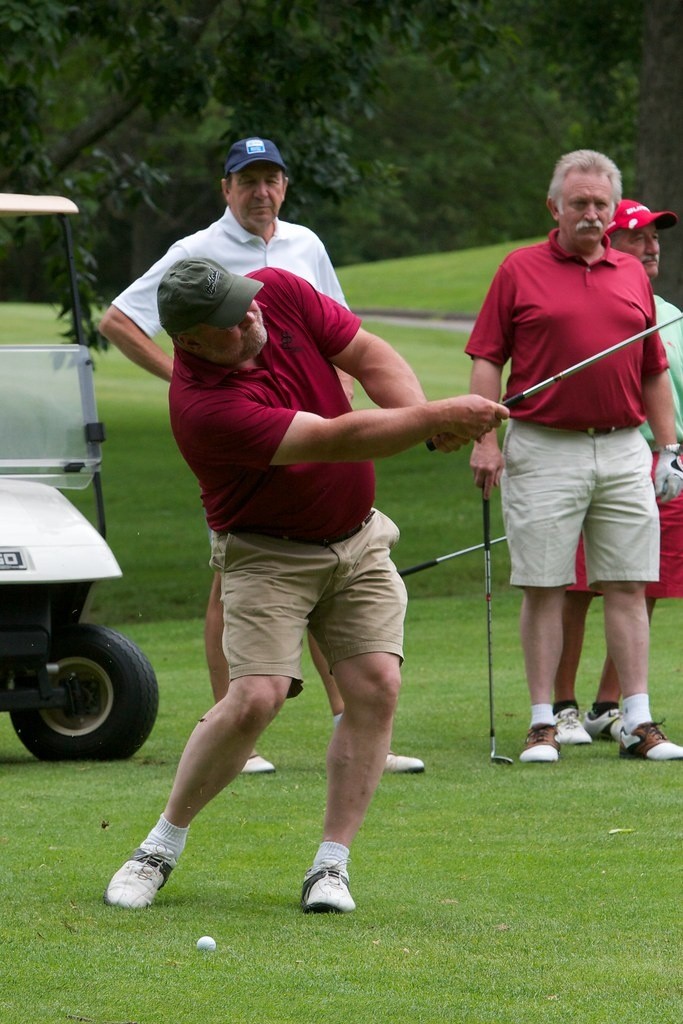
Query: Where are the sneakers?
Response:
[299,857,358,915]
[619,718,683,761]
[554,708,593,745]
[519,720,562,762]
[584,708,624,743]
[104,840,178,908]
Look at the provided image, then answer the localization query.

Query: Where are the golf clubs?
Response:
[479,476,513,764]
[423,312,682,452]
[397,534,510,580]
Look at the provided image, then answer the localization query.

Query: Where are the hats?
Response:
[225,137,289,173]
[156,258,263,337]
[604,200,676,235]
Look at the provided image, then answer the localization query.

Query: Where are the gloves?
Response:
[655,444,683,503]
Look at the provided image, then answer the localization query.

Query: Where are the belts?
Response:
[583,428,610,434]
[275,511,376,547]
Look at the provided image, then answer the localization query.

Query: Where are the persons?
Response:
[96,137,430,775]
[462,146,683,764]
[105,254,511,911]
[551,198,683,743]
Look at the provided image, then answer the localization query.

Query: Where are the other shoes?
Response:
[384,753,425,773]
[242,755,276,773]
